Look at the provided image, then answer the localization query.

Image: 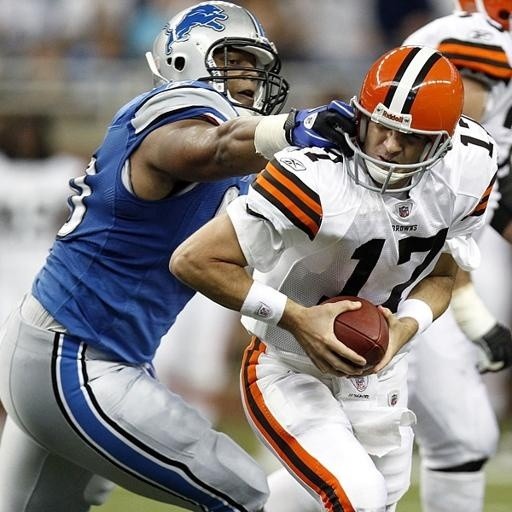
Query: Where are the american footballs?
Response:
[319,296,390,371]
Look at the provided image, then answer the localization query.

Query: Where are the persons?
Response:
[1,0,360,512]
[167,42,498,506]
[382,0,511,509]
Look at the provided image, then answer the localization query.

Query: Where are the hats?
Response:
[345,44,464,191]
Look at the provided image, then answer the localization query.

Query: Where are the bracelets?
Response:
[395,298,433,345]
[241,281,289,328]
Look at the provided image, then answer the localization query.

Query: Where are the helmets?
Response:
[456,1,512,32]
[145,0,289,116]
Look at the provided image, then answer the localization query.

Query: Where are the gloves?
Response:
[282,99,360,159]
[473,320,512,377]
